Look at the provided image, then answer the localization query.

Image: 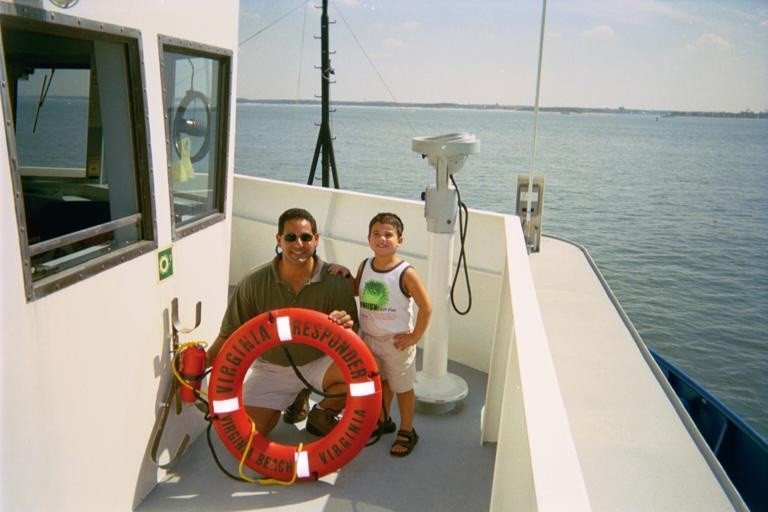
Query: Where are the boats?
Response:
[0,0,768,512]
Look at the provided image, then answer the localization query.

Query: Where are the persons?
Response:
[177,208,360,438]
[328,212,433,458]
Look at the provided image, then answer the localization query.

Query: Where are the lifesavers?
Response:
[209,309,382,482]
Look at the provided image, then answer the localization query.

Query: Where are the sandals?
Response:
[390,427,418,456]
[365,416,395,446]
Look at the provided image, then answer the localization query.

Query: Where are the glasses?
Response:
[284,233,313,242]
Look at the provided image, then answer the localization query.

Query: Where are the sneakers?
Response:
[306,404,338,436]
[283,389,312,423]
